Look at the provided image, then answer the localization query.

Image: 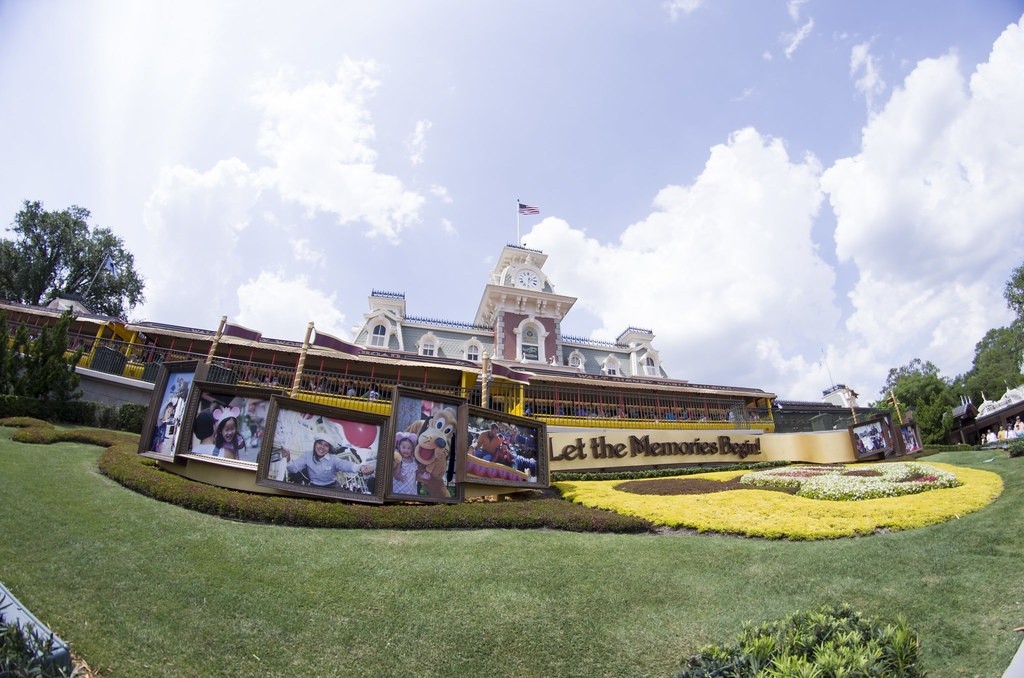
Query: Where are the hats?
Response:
[1016,416,1020,420]
[1007,423,1013,426]
[313,433,337,449]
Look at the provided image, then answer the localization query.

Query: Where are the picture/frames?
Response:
[848,411,925,462]
[135,358,552,505]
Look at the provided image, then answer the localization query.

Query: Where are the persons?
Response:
[467,424,535,466]
[154,378,189,452]
[193,404,245,460]
[981,416,1024,445]
[555,407,761,420]
[282,433,376,491]
[393,431,432,495]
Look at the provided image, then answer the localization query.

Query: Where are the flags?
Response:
[519,204,540,215]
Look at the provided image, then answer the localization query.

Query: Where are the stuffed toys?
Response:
[393,407,457,497]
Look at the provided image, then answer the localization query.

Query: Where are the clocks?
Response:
[519,272,538,288]
